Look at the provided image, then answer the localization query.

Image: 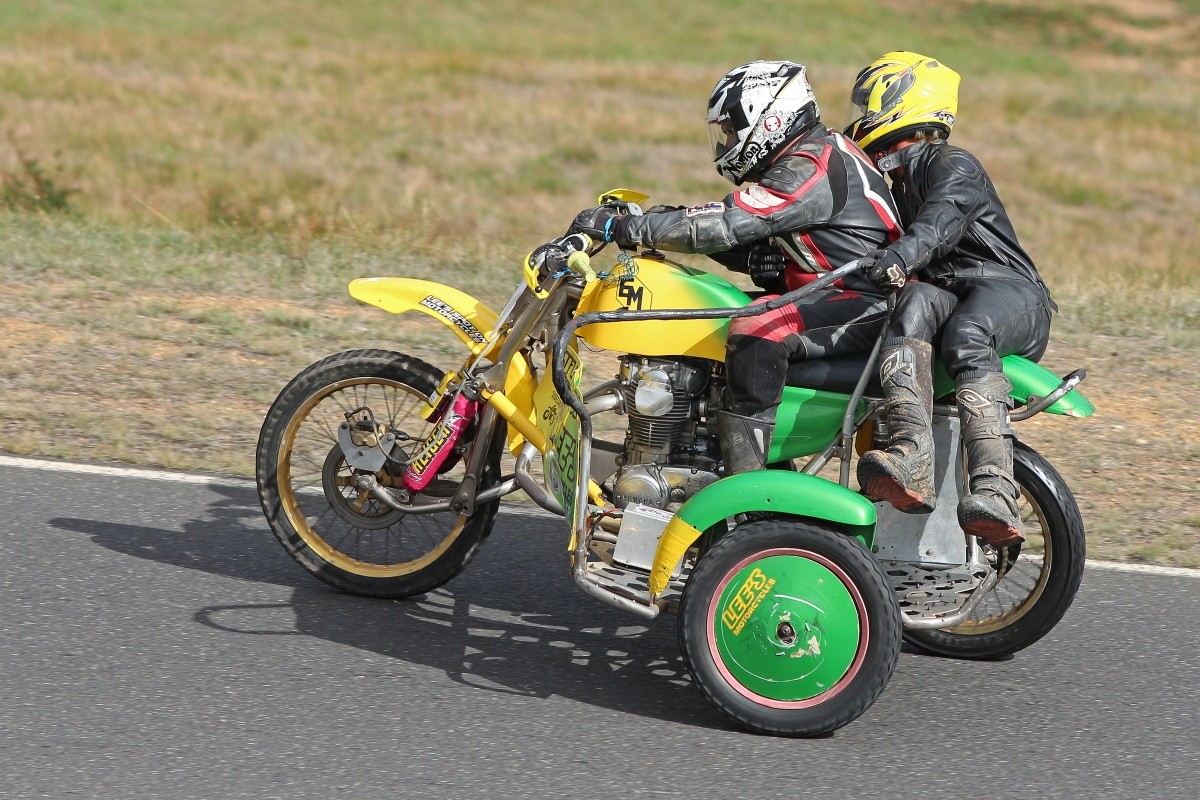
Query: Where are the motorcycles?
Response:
[254,187,1097,739]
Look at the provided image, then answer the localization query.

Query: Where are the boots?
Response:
[855,337,936,515]
[719,409,771,475]
[953,369,1026,549]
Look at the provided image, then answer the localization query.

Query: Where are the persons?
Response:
[859,50,1057,550]
[570,59,918,523]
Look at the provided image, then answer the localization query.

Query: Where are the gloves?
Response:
[864,248,908,296]
[564,208,618,246]
[608,199,645,221]
[747,245,787,288]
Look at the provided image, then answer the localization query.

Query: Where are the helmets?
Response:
[840,50,961,152]
[706,57,821,186]
